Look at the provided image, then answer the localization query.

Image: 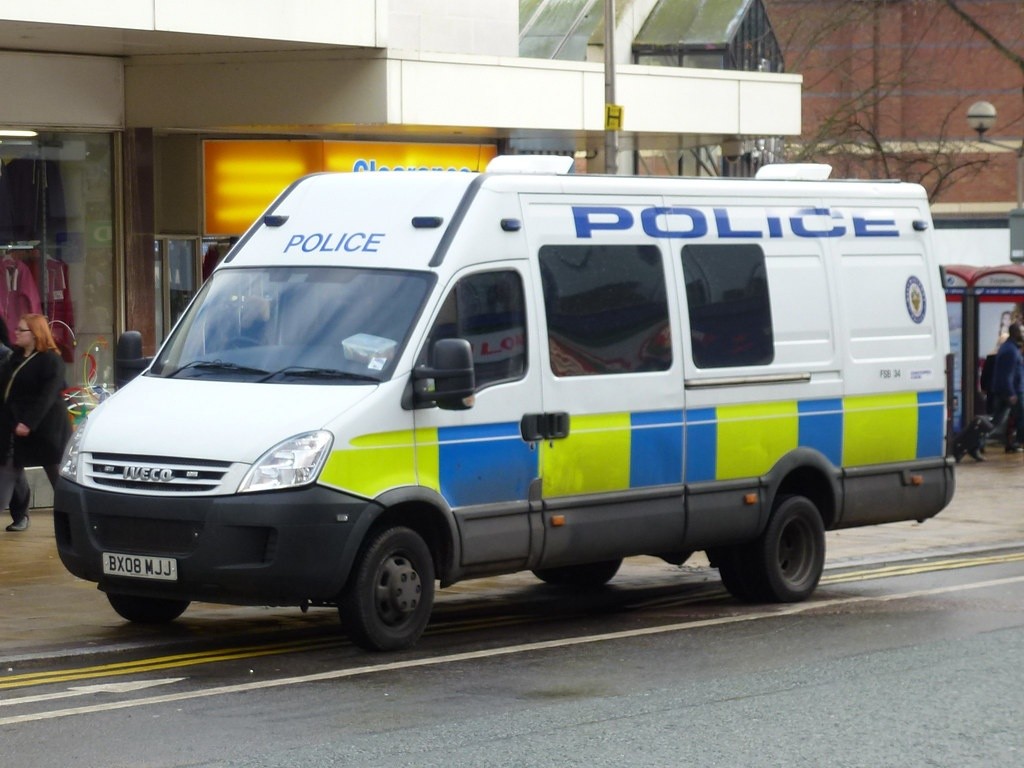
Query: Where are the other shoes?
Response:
[967,447,986,462]
[6,514,30,531]
[1005,442,1023,454]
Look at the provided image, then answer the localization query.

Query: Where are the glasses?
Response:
[14,327,32,334]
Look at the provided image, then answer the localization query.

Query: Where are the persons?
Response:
[0,313,75,531]
[967,322,1024,460]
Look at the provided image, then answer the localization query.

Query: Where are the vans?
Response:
[53,155,957,655]
